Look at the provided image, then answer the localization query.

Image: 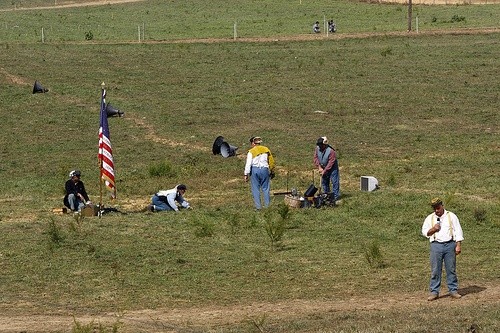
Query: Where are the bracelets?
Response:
[244,175,248,176]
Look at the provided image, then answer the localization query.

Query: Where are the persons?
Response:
[421,198,465,301]
[313,21,321,33]
[313,136,340,202]
[63,170,92,214]
[149,183,194,213]
[328,20,337,32]
[243,136,277,211]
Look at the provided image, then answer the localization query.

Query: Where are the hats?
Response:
[250,136,263,144]
[69,170,81,177]
[316,138,327,146]
[431,198,443,207]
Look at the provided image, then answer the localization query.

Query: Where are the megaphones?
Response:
[212,136,238,158]
[106,101,124,117]
[33,80,48,94]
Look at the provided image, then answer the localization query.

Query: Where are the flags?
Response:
[96,81,118,201]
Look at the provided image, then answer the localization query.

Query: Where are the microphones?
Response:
[437,218,440,231]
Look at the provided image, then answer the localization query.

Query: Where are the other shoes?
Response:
[449,292,462,299]
[150,205,154,213]
[427,293,440,301]
